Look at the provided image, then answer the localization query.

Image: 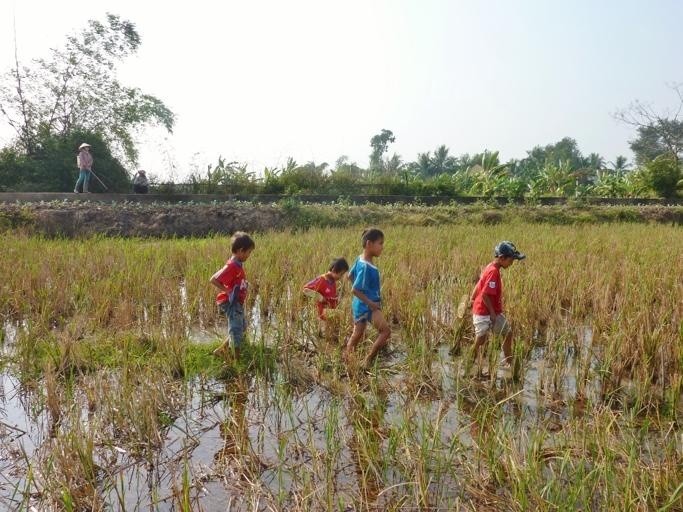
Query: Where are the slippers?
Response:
[229,285,240,307]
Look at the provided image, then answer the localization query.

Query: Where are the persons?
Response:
[134,170,150,194]
[302,255,348,342]
[74,142,94,194]
[472,241,527,380]
[345,228,391,371]
[209,230,255,364]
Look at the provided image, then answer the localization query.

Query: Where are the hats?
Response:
[494,241,526,260]
[79,143,92,150]
